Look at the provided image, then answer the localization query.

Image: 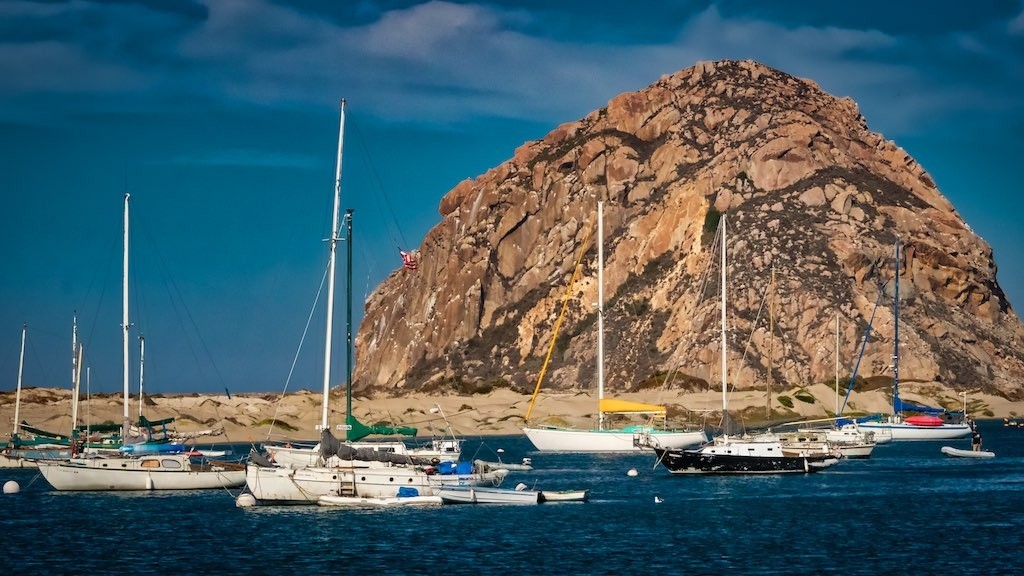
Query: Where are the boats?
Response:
[245,96,591,507]
[632,211,995,475]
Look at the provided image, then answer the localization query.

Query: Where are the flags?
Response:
[397,248,418,270]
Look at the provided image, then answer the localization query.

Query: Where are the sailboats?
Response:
[0,194,249,491]
[522,200,711,456]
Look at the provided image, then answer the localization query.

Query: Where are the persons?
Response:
[79,443,84,458]
[971,429,982,452]
[72,444,77,457]
